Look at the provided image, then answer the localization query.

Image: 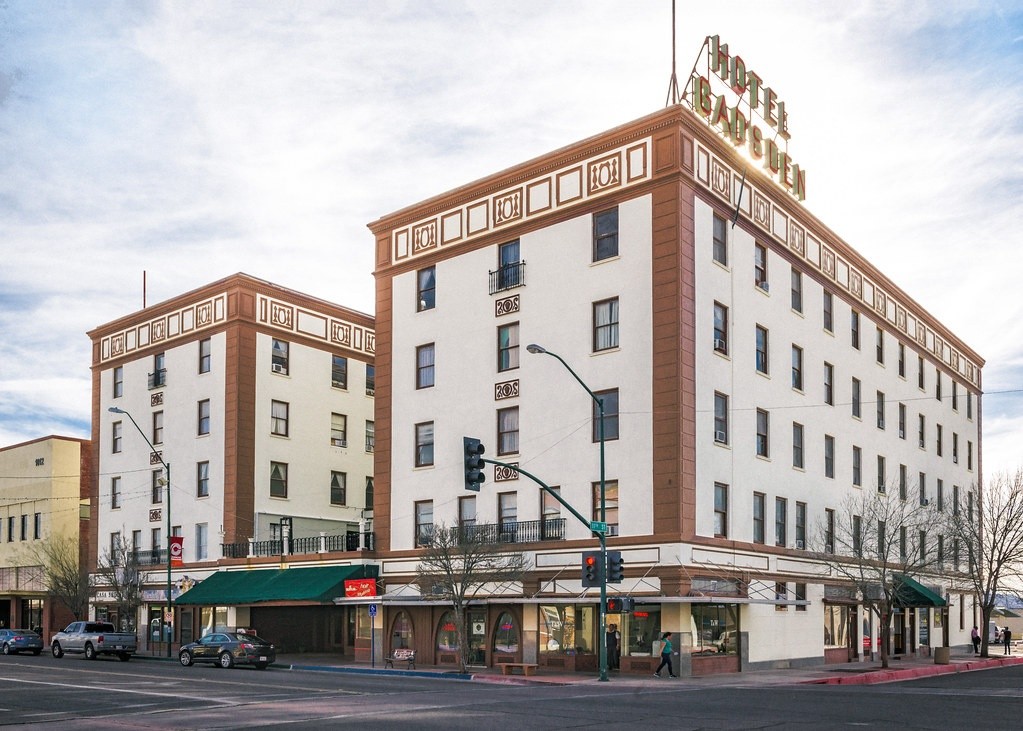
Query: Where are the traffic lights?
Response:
[607,551,624,584]
[606,597,623,611]
[582,551,602,587]
[463,436,486,491]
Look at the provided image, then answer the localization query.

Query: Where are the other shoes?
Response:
[669,675,677,677]
[654,673,660,678]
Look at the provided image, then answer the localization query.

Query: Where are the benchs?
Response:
[384,649,418,670]
[495,662,538,676]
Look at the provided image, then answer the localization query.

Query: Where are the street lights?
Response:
[526,344,608,682]
[108,407,171,658]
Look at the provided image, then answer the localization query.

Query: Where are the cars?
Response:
[178,631,276,671]
[0,629,44,656]
[990,625,1005,644]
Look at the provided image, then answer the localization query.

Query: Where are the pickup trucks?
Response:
[50,620,138,662]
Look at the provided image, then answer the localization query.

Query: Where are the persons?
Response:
[1003,626,1012,655]
[605,624,622,670]
[654,632,678,679]
[971,626,980,654]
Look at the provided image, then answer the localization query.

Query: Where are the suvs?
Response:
[690,629,738,657]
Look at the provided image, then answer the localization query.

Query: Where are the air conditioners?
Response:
[272,363,282,372]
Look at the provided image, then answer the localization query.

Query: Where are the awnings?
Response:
[892,572,947,605]
[174,564,364,605]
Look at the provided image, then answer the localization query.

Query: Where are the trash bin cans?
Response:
[934,646,950,665]
